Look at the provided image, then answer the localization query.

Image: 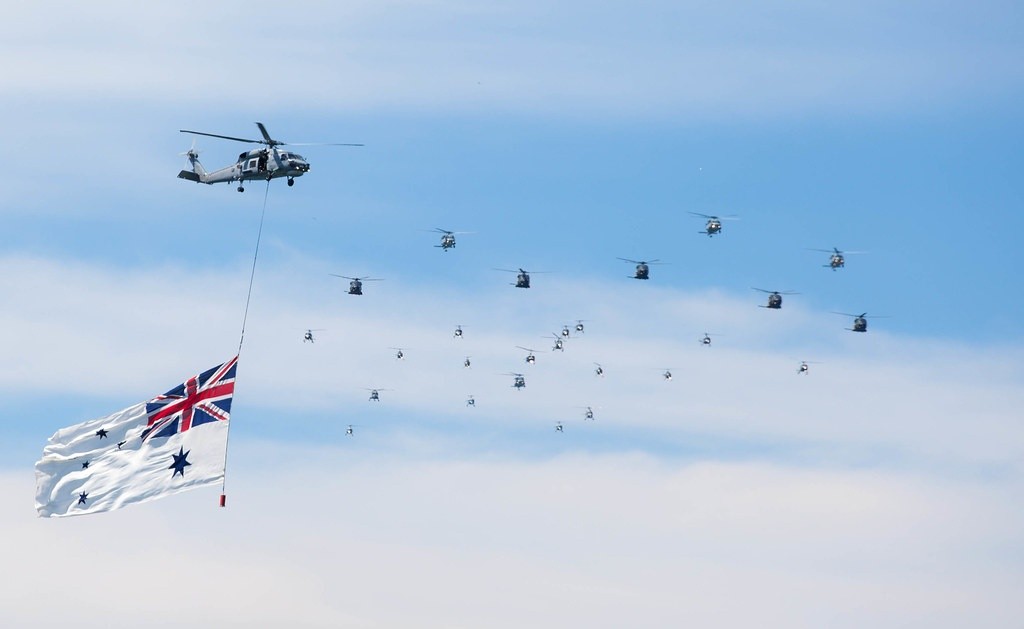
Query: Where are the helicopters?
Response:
[388,346,411,360]
[175,123,367,193]
[330,269,384,300]
[490,265,554,290]
[684,210,739,238]
[749,286,802,311]
[366,387,389,402]
[451,324,468,338]
[806,246,865,271]
[460,318,603,436]
[661,366,679,381]
[429,227,474,252]
[794,361,815,375]
[828,308,895,334]
[618,256,672,280]
[698,331,719,346]
[302,328,322,344]
[342,423,362,438]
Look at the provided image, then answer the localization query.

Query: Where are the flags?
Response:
[34,355,237,516]
